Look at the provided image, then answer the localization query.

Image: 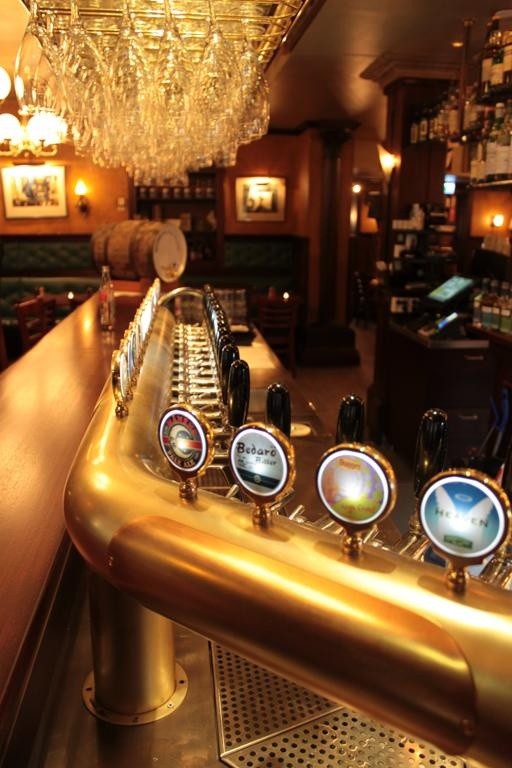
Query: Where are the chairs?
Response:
[259,294,299,368]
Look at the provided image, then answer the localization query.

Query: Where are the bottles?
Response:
[409,10,512,183]
[471,277,511,333]
[139,177,214,199]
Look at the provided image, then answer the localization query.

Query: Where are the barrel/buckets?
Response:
[91,218,189,285]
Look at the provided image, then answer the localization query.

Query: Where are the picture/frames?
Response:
[1,165,68,221]
[235,175,287,223]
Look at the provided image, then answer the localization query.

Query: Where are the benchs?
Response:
[1,278,100,327]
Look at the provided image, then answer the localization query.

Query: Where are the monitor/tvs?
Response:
[421,274,478,308]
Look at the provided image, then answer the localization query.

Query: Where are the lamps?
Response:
[75,179,89,212]
[0,68,64,158]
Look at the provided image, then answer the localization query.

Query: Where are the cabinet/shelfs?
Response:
[129,167,226,277]
